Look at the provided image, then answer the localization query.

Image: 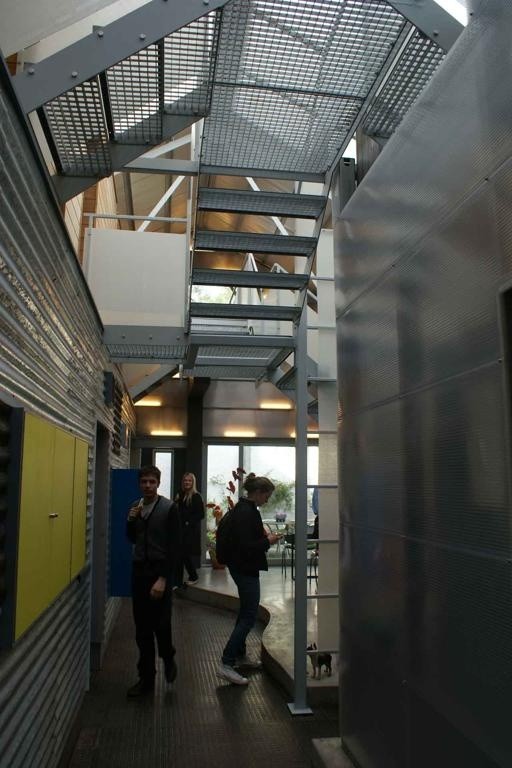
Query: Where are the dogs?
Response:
[306,642,333,680]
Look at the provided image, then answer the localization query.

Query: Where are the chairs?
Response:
[281,534,317,585]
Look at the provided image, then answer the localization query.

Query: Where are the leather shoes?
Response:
[127,679,155,698]
[165,657,177,683]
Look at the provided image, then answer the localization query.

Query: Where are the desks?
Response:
[264,521,295,553]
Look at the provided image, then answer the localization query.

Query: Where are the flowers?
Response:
[209,530,217,547]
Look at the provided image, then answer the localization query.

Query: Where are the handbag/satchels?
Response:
[216,510,236,565]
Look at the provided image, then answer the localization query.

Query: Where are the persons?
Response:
[215,472,283,684]
[124,464,180,697]
[171,472,205,585]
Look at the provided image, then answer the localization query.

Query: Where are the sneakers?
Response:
[215,658,264,686]
[184,579,198,586]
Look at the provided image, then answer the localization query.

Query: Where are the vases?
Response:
[210,547,226,570]
